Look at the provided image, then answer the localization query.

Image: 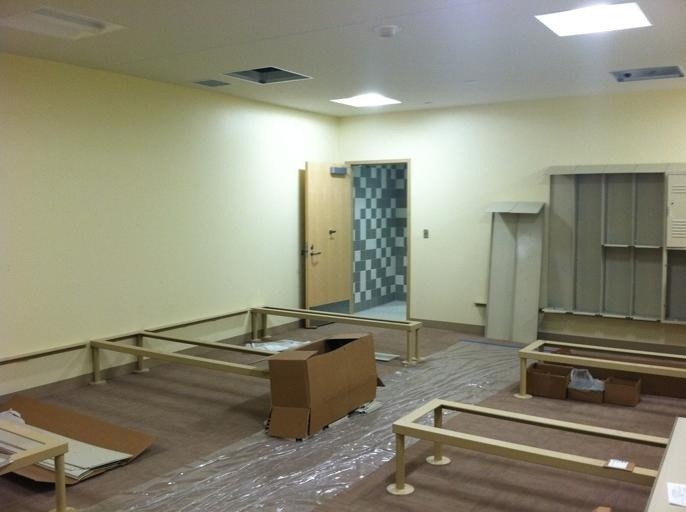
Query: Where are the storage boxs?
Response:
[605,372,643,407]
[567,375,605,403]
[527,361,574,400]
[265,332,386,443]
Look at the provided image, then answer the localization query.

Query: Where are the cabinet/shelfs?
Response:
[536,162,686,363]
